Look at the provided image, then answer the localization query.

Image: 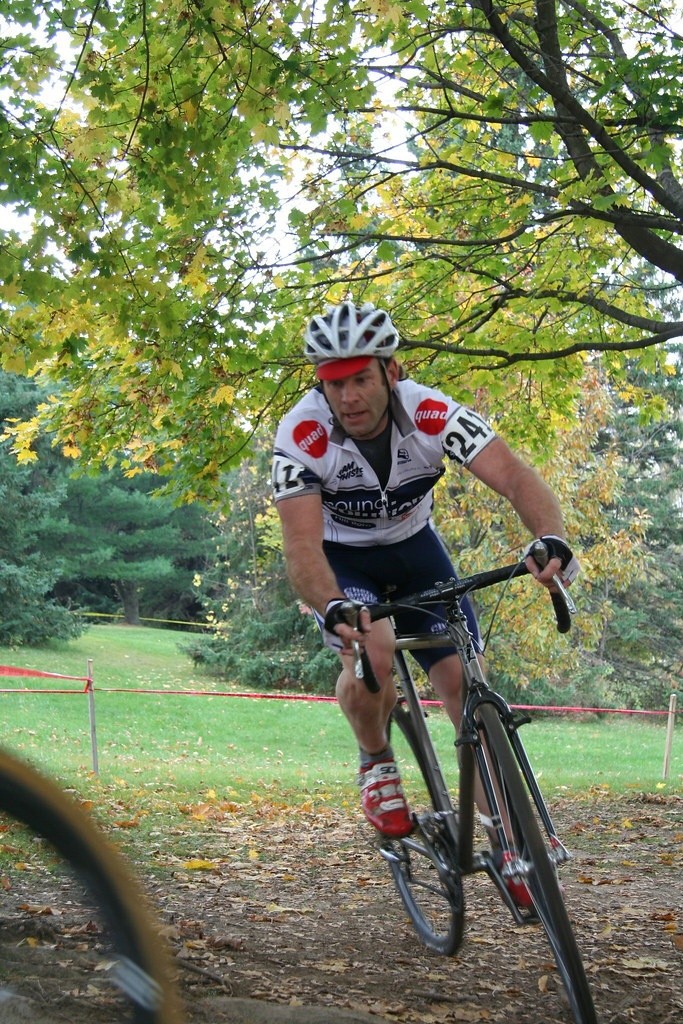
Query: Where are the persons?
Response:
[267,302,579,920]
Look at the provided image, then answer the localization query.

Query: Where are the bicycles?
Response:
[344,539,596,1023]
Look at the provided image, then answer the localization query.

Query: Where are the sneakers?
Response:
[498,851,533,906]
[359,757,413,838]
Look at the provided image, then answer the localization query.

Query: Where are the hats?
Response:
[316,313,388,381]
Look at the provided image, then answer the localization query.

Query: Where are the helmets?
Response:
[304,301,399,358]
